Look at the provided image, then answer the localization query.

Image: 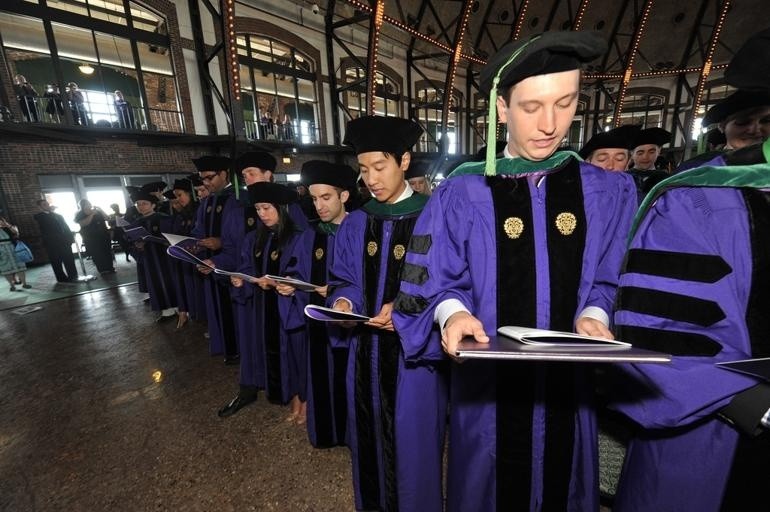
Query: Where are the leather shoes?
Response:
[155,312,177,322]
[142,297,151,302]
[286,411,306,424]
[219,396,257,416]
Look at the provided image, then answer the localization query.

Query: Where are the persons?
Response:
[260,111,295,141]
[124,151,352,450]
[34,199,79,282]
[13,73,89,126]
[578,32,768,508]
[391,29,639,511]
[4,217,32,291]
[76,200,123,271]
[323,117,509,507]
[113,90,135,128]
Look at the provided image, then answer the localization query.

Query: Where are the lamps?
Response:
[78,62,94,75]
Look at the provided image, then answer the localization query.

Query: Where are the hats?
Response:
[301,160,355,189]
[342,116,423,155]
[577,124,673,161]
[479,32,603,176]
[192,152,296,204]
[126,179,191,202]
[698,29,770,156]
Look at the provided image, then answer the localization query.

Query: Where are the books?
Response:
[116,216,130,227]
[162,233,201,245]
[123,226,151,240]
[166,245,213,268]
[456,326,670,362]
[305,304,370,322]
[141,235,165,244]
[264,274,323,293]
[214,269,259,283]
